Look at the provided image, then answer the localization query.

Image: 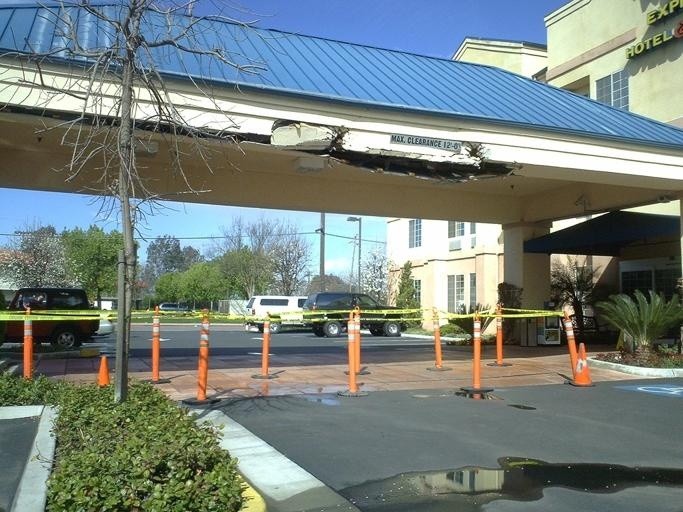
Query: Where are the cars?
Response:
[89,306,114,337]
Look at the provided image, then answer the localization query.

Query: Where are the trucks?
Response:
[91,300,113,310]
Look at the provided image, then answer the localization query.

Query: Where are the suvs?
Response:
[2,283,98,350]
[304,291,407,337]
[157,303,189,314]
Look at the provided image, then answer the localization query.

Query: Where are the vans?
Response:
[245,295,310,333]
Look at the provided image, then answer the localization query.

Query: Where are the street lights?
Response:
[347,214,362,293]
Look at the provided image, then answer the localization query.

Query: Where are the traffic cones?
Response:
[570,341,594,387]
[93,355,111,388]
